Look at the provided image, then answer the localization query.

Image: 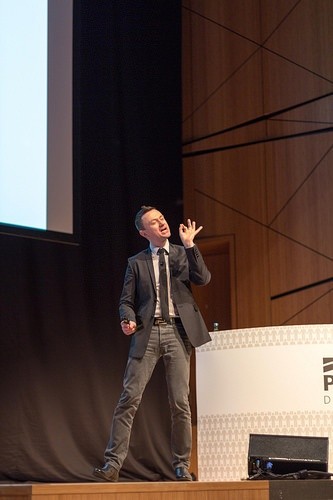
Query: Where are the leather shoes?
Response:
[94,464,119,482]
[176,467,192,481]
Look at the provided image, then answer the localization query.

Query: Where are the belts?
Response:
[153,317,181,325]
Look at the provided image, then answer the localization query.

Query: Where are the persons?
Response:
[93,205,212,481]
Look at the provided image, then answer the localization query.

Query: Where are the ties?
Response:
[157,248,169,321]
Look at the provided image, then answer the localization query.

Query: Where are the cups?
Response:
[213,322,219,331]
